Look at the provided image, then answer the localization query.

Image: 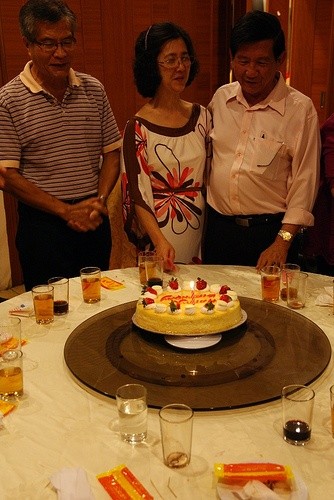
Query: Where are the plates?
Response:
[215,453,308,500]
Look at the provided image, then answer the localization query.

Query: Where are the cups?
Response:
[0,317,23,361]
[0,347,24,398]
[158,403,195,468]
[31,284,54,324]
[259,266,281,303]
[329,384,334,438]
[285,272,309,310]
[279,263,301,300]
[80,267,101,304]
[282,384,316,447]
[47,277,69,314]
[115,383,150,442]
[138,254,163,289]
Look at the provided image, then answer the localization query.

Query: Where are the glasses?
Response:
[158,56,194,66]
[31,36,77,50]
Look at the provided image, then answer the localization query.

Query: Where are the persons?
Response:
[119,22,212,271]
[0,0,123,295]
[200,10,321,275]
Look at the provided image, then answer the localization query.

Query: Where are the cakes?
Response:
[134,277,244,334]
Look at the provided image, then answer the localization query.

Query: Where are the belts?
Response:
[232,213,282,228]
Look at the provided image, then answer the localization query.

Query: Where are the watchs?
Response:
[277,229,295,242]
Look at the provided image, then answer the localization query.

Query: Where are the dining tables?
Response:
[0,263,334,499]
